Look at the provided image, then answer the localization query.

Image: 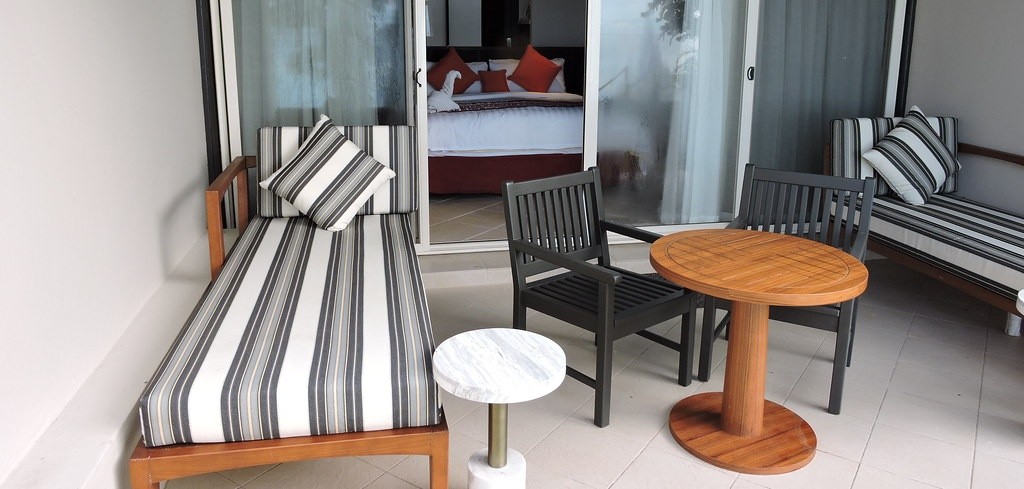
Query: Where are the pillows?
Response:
[507,44,562,94]
[862,104,963,206]
[255,123,418,217]
[488,57,566,92]
[479,69,510,93]
[257,113,398,237]
[831,117,959,199]
[427,46,479,94]
[426,60,488,96]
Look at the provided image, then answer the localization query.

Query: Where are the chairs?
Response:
[697,162,878,415]
[501,167,699,426]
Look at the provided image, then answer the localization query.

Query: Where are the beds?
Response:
[820,144,1024,318]
[424,43,583,194]
[128,155,449,489]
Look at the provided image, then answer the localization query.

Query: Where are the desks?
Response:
[647,227,868,475]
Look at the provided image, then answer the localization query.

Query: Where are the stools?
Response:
[430,327,566,489]
